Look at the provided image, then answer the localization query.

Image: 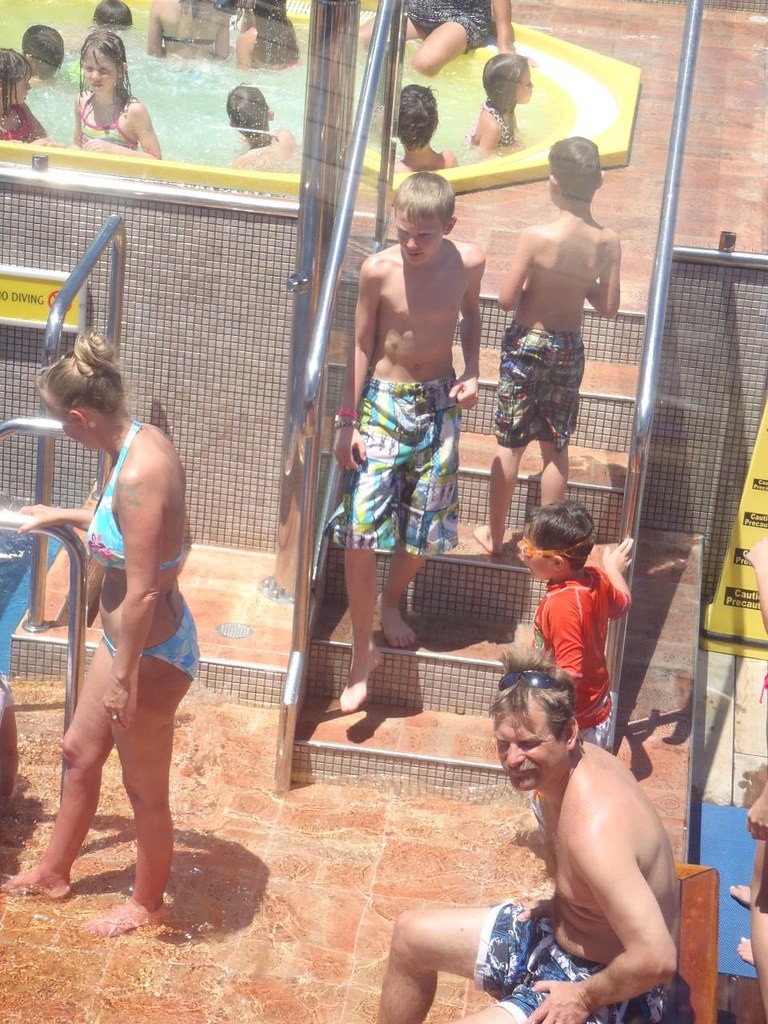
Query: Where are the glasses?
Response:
[498,670,563,691]
[516,537,535,559]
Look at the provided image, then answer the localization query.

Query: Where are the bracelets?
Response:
[339,408,358,419]
[335,420,360,429]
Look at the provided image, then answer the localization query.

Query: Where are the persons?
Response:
[0,330,199,937]
[72,31,162,161]
[465,53,534,149]
[0,48,65,147]
[230,0,302,72]
[94,0,136,26]
[22,25,65,82]
[517,500,634,835]
[730,538,768,1024]
[358,0,539,77]
[147,0,230,62]
[474,136,622,556]
[323,172,486,713]
[376,646,679,1023]
[0,670,20,798]
[225,83,300,174]
[396,84,459,173]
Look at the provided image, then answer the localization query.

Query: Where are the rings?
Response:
[111,715,118,720]
